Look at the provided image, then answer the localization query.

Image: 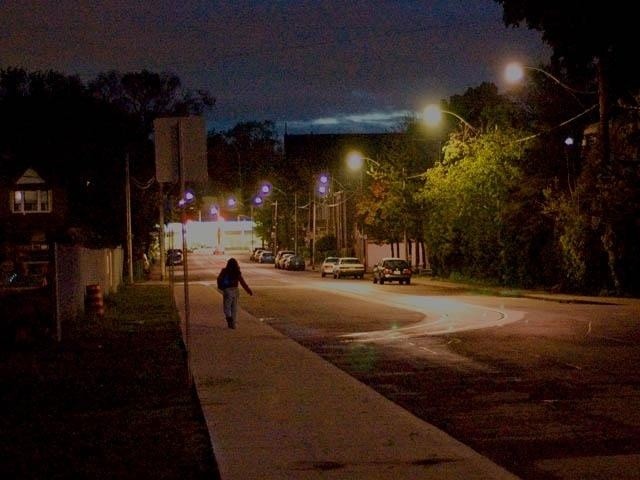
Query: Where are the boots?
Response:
[225,316,235,330]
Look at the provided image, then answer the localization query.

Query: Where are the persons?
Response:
[217,258,253,329]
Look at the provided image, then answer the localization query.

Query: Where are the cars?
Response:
[167,248,183,265]
[249,248,305,272]
[321,257,412,284]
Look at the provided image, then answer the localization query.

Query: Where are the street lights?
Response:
[254,184,299,254]
[211,205,224,255]
[422,105,484,137]
[318,177,348,249]
[347,151,408,261]
[158,176,204,282]
[229,198,255,249]
[504,60,610,179]
